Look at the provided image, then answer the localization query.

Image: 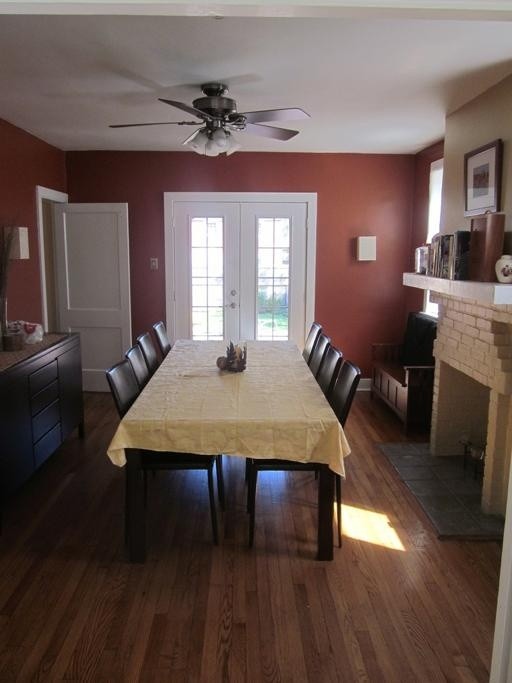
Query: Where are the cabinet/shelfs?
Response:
[0,331,86,508]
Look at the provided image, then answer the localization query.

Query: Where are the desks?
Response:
[121,337,339,566]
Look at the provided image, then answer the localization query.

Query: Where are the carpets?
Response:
[372,441,506,543]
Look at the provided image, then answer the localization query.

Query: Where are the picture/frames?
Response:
[461,138,503,218]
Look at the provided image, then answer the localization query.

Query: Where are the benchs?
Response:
[367,311,436,438]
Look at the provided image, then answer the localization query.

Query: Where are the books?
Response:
[412,229,473,280]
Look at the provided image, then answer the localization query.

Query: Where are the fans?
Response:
[107,82,314,159]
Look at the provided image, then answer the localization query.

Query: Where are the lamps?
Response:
[187,129,244,157]
